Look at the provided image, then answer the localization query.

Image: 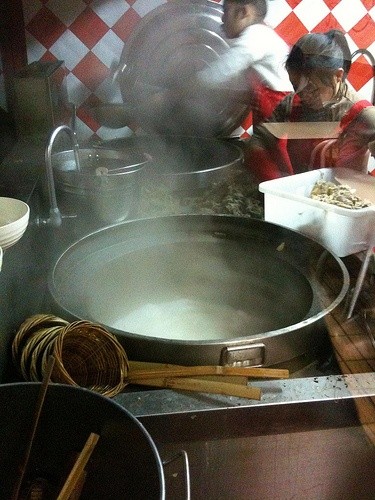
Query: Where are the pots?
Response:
[49,147,153,227]
[46,212,351,368]
[0,381,194,500]
[93,134,245,192]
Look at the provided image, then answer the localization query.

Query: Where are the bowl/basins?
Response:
[0,196,31,249]
[0,247,4,272]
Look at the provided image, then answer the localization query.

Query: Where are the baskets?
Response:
[12,312,129,397]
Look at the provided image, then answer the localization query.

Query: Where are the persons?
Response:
[243,29,375,181]
[93,0,296,136]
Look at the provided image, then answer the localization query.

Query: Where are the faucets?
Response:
[43,124,82,230]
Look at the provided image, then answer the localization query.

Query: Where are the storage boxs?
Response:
[258,167,375,257]
